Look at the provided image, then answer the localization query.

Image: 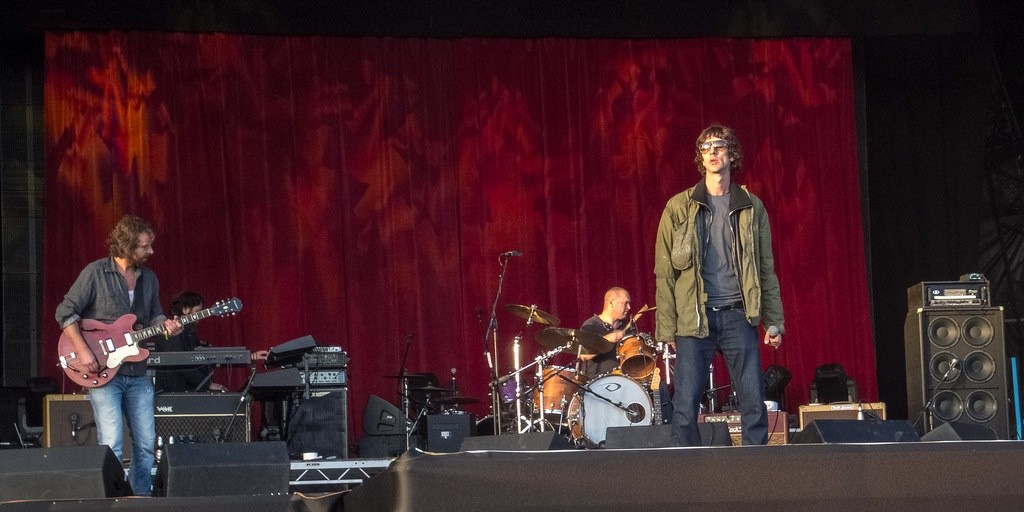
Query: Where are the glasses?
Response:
[698,140,730,155]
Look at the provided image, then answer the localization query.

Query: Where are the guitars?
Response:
[651,367,661,426]
[58,297,245,386]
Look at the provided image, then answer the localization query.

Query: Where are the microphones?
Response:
[857,405,865,420]
[501,251,523,256]
[629,313,635,330]
[69,413,79,438]
[450,368,456,381]
[623,407,641,418]
[768,325,779,354]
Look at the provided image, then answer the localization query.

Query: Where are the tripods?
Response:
[488,315,577,434]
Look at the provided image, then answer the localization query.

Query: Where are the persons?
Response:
[155,292,269,392]
[653,125,786,448]
[55,215,185,497]
[579,287,673,425]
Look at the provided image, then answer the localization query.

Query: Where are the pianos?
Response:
[145,346,251,395]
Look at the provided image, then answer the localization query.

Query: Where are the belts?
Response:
[706,301,743,312]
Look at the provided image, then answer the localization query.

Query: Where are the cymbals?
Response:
[537,327,612,354]
[432,395,477,402]
[504,302,561,326]
[410,384,451,391]
[387,374,429,381]
[637,306,657,314]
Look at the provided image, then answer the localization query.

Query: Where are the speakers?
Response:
[274,386,348,458]
[605,421,732,450]
[42,393,135,463]
[0,445,134,502]
[357,394,424,459]
[459,432,574,451]
[697,411,789,446]
[151,442,291,496]
[789,307,1010,444]
[153,393,251,465]
[427,414,476,454]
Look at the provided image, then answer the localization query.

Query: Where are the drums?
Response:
[567,372,655,445]
[616,333,656,379]
[534,364,590,417]
